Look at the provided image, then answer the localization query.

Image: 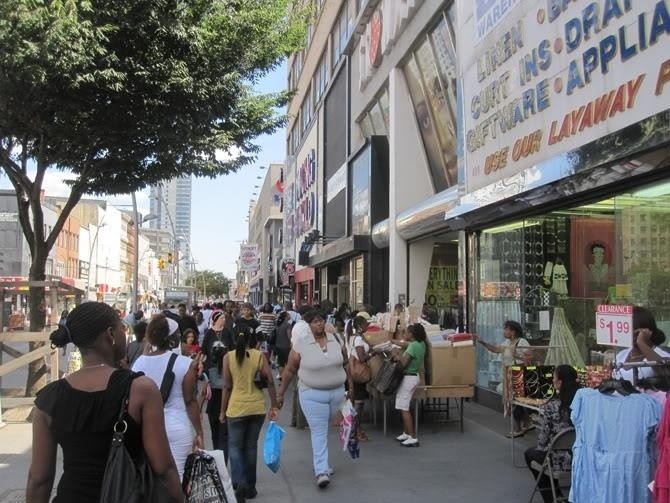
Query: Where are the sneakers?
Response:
[244,486,259,499]
[316,474,330,489]
[234,486,247,503]
[400,435,420,447]
[396,431,410,442]
[327,463,334,475]
[355,434,369,442]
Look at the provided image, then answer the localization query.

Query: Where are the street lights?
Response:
[173,234,186,285]
[87,219,106,294]
[123,211,160,321]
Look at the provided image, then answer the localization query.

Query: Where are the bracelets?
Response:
[368,353,371,357]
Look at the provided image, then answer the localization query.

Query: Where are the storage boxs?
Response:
[362,318,477,387]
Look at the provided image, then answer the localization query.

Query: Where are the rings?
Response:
[642,329,645,332]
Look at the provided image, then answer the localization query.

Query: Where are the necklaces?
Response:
[627,345,656,362]
[509,369,555,400]
[80,363,107,370]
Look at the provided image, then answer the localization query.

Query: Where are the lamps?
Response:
[304,229,342,245]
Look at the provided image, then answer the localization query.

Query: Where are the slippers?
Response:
[506,432,524,439]
[521,425,536,432]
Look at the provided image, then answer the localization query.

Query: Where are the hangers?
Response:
[594,357,670,396]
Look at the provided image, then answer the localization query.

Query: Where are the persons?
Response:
[478,320,538,438]
[25,302,183,501]
[610,306,669,384]
[218,325,279,501]
[524,364,584,502]
[131,317,205,485]
[392,323,428,446]
[124,298,384,466]
[277,308,361,488]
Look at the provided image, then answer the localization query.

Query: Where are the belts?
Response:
[403,372,418,377]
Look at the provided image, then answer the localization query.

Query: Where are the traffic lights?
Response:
[160,257,166,270]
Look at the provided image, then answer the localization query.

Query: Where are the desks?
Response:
[366,383,475,442]
[509,396,548,468]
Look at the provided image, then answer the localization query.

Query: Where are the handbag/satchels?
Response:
[262,416,287,475]
[252,352,269,390]
[347,398,361,459]
[338,391,353,452]
[369,354,404,396]
[184,446,238,503]
[347,354,372,384]
[181,450,229,503]
[266,329,276,346]
[98,417,170,503]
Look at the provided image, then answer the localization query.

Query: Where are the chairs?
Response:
[528,427,576,502]
